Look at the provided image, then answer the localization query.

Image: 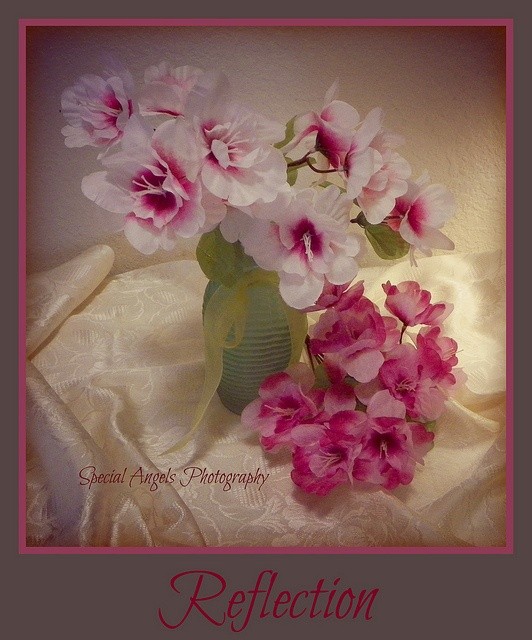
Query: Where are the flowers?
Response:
[60,63,459,495]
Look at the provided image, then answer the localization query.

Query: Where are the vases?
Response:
[201,262,308,415]
